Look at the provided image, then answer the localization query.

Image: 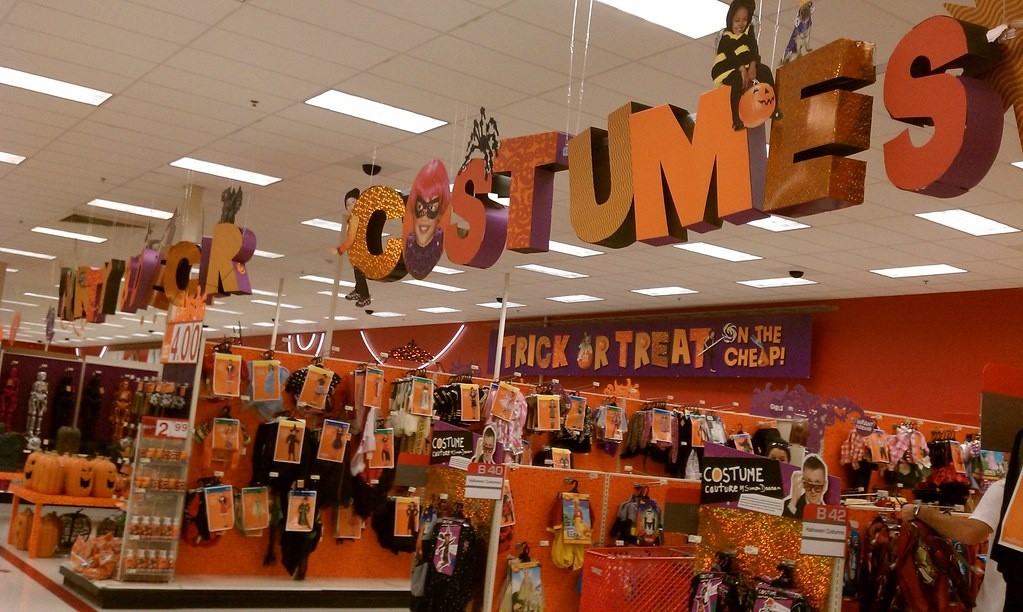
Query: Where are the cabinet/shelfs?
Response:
[114,422,188,582]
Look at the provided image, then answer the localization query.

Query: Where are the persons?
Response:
[216,492,233,528]
[764,438,791,464]
[399,159,450,279]
[902,452,1022,612]
[474,430,498,466]
[709,0,783,130]
[343,185,372,307]
[783,455,831,520]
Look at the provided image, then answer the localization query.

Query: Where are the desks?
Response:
[7,483,124,560]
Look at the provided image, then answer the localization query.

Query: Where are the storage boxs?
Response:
[578,545,697,612]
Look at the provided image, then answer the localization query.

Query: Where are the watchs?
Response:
[912,505,921,522]
